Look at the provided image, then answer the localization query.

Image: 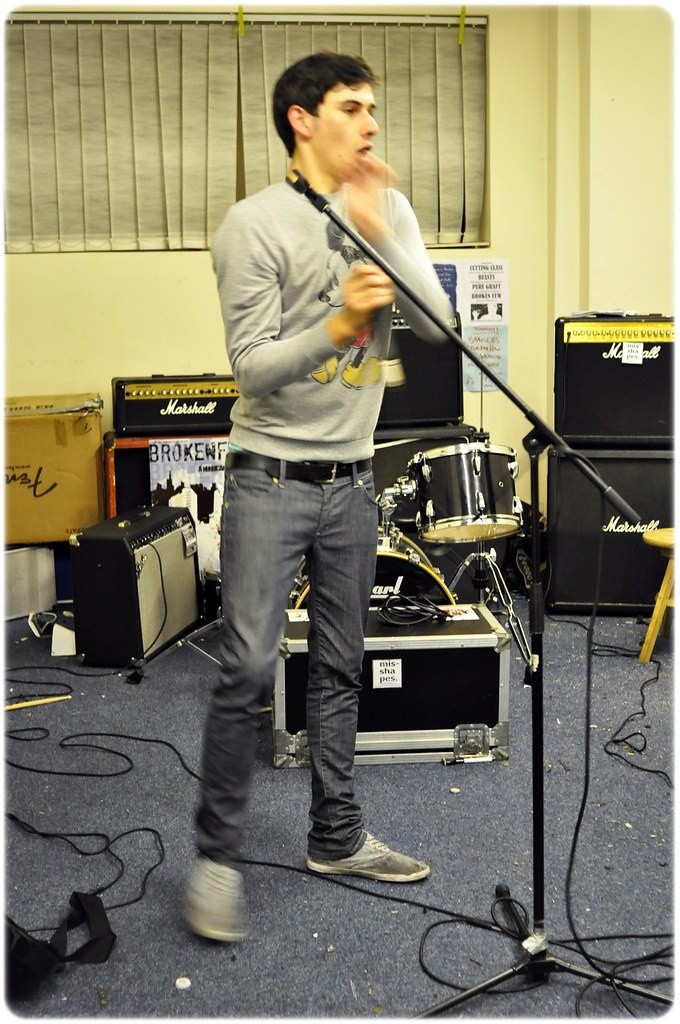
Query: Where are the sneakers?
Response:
[306,832,431,882]
[184,854,248,942]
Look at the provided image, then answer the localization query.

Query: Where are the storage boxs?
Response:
[5,392,107,546]
[270,602,511,770]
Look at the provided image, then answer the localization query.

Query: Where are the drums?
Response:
[285,525,456,606]
[406,442,523,543]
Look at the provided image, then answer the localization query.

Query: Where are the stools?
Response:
[637,527,675,661]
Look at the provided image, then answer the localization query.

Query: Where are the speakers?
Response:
[364,311,486,604]
[68,431,231,670]
[547,312,673,617]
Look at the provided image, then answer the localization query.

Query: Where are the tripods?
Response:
[286,167,673,1023]
[446,542,534,686]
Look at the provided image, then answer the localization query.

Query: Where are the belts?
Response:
[225,453,373,484]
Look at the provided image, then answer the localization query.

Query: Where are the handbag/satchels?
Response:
[6,890,117,1002]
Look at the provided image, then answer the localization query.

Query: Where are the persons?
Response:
[178,51,454,947]
